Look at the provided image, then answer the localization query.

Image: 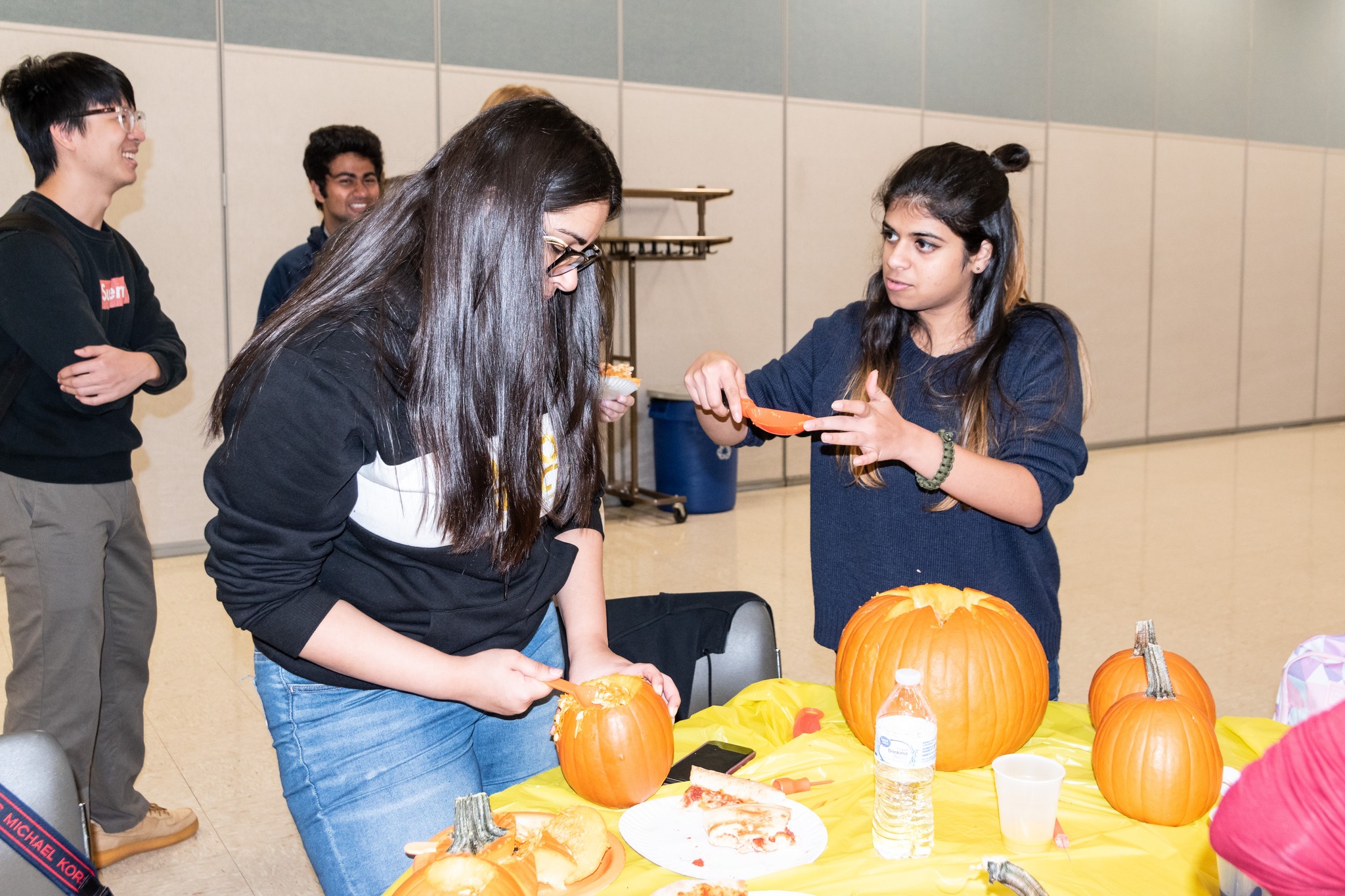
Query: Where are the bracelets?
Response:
[914,427,955,492]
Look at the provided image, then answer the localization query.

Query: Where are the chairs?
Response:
[0,729,92,895]
[554,593,781,724]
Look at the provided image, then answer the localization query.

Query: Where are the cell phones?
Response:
[661,741,756,785]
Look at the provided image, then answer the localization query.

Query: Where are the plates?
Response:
[618,793,828,881]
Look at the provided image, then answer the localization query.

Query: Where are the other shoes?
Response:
[90,803,199,870]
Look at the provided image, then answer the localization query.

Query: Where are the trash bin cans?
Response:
[646,390,737,514]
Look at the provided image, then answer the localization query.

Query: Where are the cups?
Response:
[993,753,1066,856]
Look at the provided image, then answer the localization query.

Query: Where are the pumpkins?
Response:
[834,583,1050,772]
[550,672,675,809]
[1090,643,1224,827]
[1088,619,1216,734]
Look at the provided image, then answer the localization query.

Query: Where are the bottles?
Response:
[873,668,938,859]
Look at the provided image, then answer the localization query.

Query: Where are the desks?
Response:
[379,677,1345,896]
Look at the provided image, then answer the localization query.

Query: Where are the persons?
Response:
[481,85,641,427]
[0,48,202,869]
[680,144,1087,699]
[200,95,682,896]
[251,118,392,337]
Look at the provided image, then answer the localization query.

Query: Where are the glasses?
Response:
[59,107,147,133]
[541,231,603,278]
